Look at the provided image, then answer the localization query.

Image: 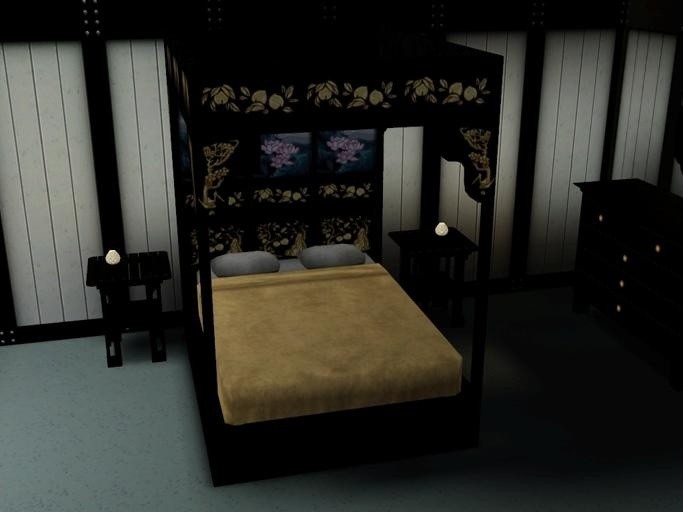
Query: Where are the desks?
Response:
[387,227,477,326]
[84,249,172,369]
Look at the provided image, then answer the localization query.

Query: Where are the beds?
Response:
[161,36,507,487]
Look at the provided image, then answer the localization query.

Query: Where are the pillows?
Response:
[209,241,366,279]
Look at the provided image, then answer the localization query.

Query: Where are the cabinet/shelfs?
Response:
[568,177,683,394]
